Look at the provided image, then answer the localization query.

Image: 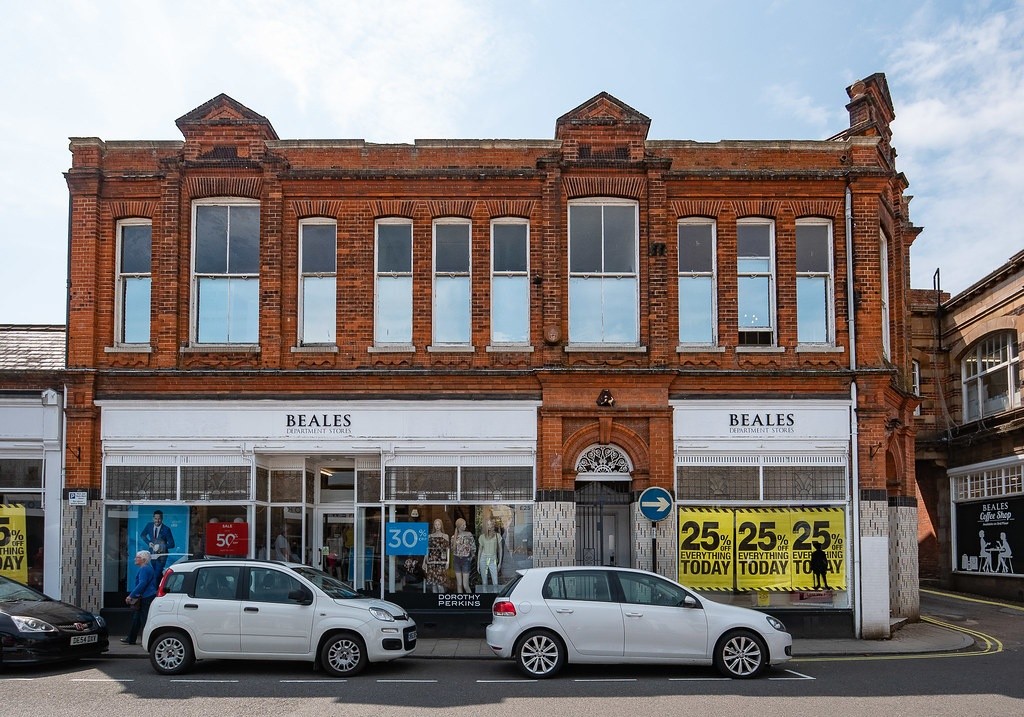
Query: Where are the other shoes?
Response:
[120,638,135,644]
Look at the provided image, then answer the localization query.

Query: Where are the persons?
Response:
[258,524,292,562]
[120,551,157,645]
[421,518,503,593]
[490,491,513,540]
[140,510,175,587]
[191,533,201,552]
[326,524,343,581]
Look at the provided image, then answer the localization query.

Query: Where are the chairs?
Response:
[194,572,276,602]
[595,580,609,600]
[547,585,554,599]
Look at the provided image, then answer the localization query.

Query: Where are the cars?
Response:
[141,559,418,677]
[485,566,792,680]
[0,575,110,671]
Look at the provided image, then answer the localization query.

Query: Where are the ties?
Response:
[155,528,157,538]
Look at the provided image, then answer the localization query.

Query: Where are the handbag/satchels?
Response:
[129,598,141,610]
